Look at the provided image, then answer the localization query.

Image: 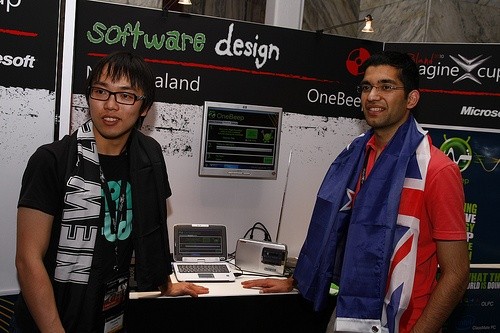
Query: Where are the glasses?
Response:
[356,83,406,93]
[89,86,147,105]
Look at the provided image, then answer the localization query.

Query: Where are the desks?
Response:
[127,258,301,300]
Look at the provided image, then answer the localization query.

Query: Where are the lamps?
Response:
[313,14,374,39]
[161,0,192,17]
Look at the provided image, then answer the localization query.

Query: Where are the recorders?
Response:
[235,150,292,276]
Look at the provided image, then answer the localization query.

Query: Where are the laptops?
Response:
[173,224,236,282]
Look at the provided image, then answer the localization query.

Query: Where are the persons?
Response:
[240,51,468,333]
[15,48,209,333]
